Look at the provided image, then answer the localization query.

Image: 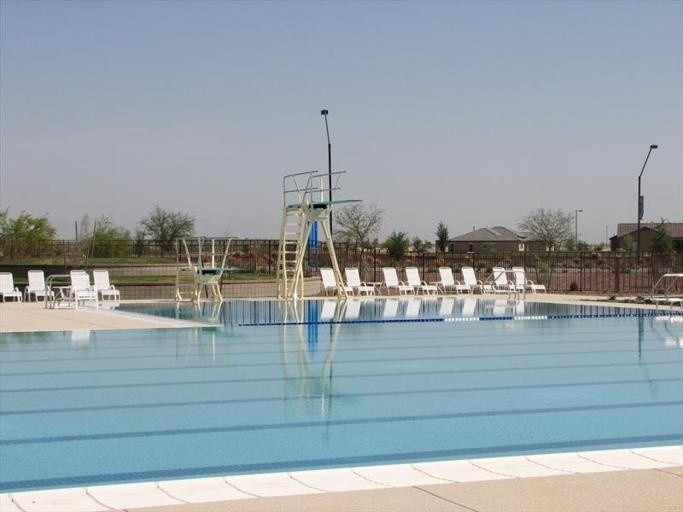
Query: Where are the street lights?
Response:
[573,209,583,250]
[318,107,332,238]
[635,144,658,260]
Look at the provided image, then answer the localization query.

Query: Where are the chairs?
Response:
[0,269,120,312]
[319,297,525,321]
[317,266,546,296]
